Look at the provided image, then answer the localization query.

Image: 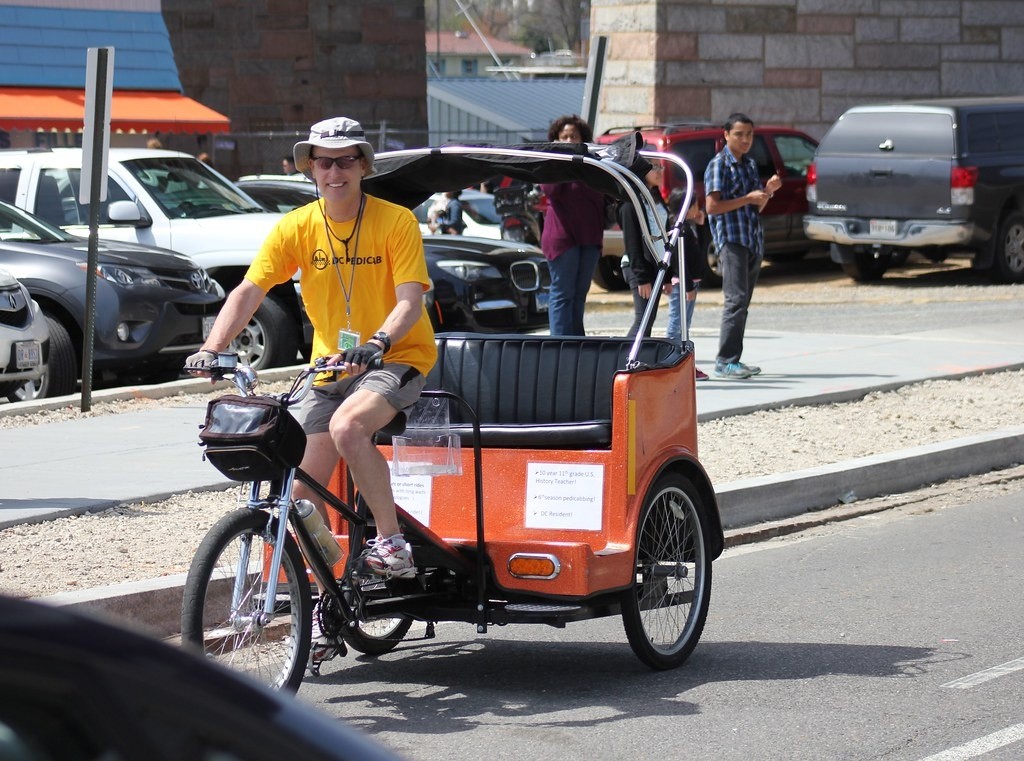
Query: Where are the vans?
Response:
[801,94,1024,286]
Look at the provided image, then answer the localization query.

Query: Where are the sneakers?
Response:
[357,535,417,578]
[308,602,342,659]
[715,360,761,379]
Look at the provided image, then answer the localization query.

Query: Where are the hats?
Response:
[293,116,379,182]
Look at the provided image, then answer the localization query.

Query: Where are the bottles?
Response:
[295,499,342,565]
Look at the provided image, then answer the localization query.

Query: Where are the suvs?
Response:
[0,146,315,371]
[592,121,832,291]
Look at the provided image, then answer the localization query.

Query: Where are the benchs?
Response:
[370,333,693,450]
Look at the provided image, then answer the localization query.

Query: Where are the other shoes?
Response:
[694,370,709,382]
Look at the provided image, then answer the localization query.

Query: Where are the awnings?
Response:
[0,86,231,134]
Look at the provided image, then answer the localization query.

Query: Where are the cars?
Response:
[0,198,227,404]
[230,178,553,335]
[411,189,502,240]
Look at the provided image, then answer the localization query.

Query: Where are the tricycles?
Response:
[179,137,726,700]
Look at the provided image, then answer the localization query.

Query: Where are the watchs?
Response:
[372,332,391,354]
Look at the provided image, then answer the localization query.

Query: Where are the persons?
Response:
[617,158,673,336]
[541,117,613,336]
[283,156,296,175]
[703,113,782,379]
[427,189,463,236]
[185,117,438,661]
[666,187,709,380]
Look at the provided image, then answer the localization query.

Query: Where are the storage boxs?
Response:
[493,184,527,213]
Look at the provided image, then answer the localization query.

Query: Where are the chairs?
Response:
[34,175,67,226]
[64,169,112,227]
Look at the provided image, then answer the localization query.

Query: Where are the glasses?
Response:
[311,155,364,170]
[651,164,665,172]
